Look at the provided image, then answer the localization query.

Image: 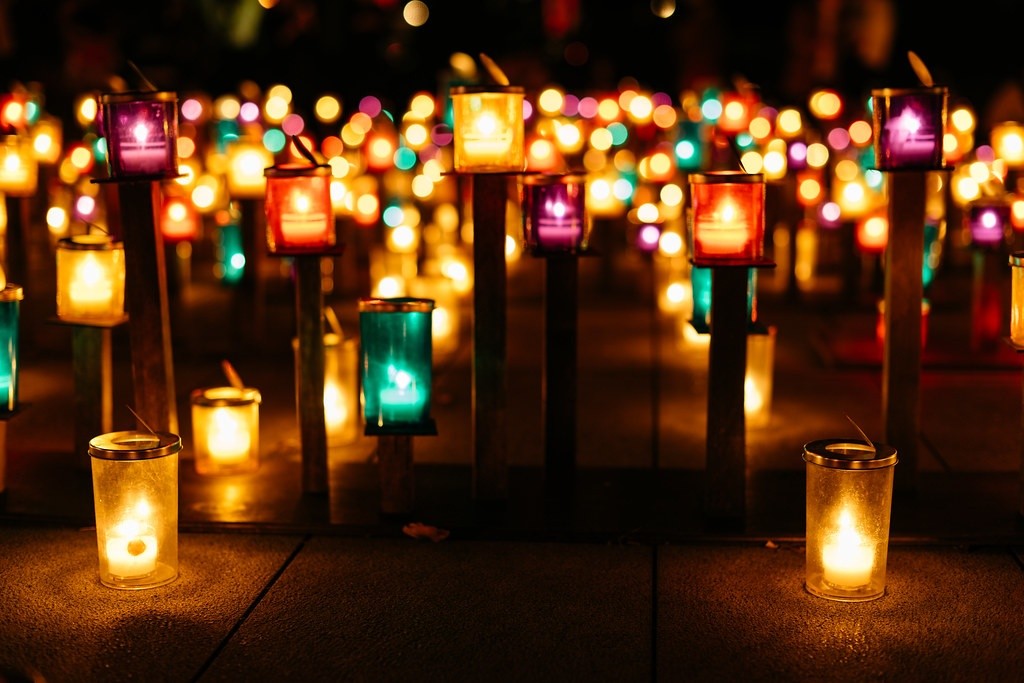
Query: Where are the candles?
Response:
[0,90,1024,476]
[88,403,181,592]
[796,418,899,601]
